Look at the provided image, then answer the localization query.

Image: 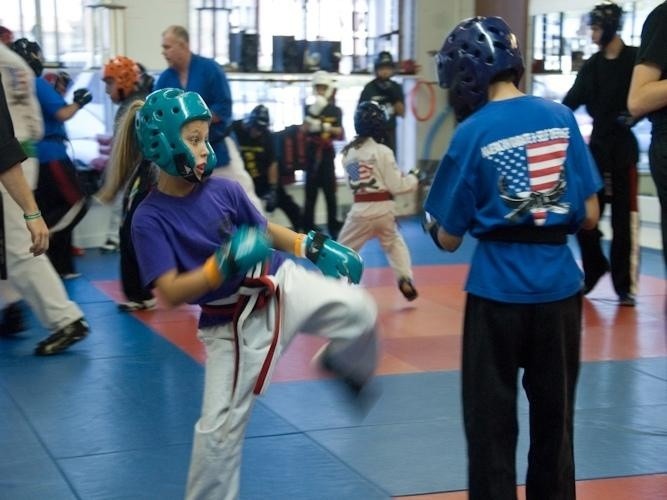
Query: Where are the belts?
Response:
[477,224,569,246]
[202,256,283,444]
[354,192,392,203]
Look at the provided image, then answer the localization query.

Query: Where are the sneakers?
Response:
[619,293,637,306]
[398,276,418,301]
[100,236,120,252]
[118,297,158,312]
[35,319,90,355]
[580,256,611,295]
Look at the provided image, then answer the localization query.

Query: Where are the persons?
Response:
[13,39,94,278]
[422,16,602,499]
[226,102,304,226]
[0,39,91,356]
[335,101,422,302]
[158,26,234,167]
[554,1,639,309]
[103,55,159,313]
[301,69,344,238]
[1,75,50,278]
[98,89,381,499]
[362,52,404,156]
[627,1,667,265]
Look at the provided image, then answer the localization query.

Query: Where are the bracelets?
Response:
[25,211,44,219]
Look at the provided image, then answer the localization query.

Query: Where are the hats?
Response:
[374,52,396,69]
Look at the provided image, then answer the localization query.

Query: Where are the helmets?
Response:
[310,71,334,98]
[242,105,270,135]
[9,38,43,77]
[435,17,525,122]
[354,101,387,134]
[135,87,218,178]
[102,56,143,104]
[587,4,623,47]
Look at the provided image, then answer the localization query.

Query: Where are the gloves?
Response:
[205,226,272,289]
[295,229,366,285]
[73,89,93,108]
[618,111,638,127]
